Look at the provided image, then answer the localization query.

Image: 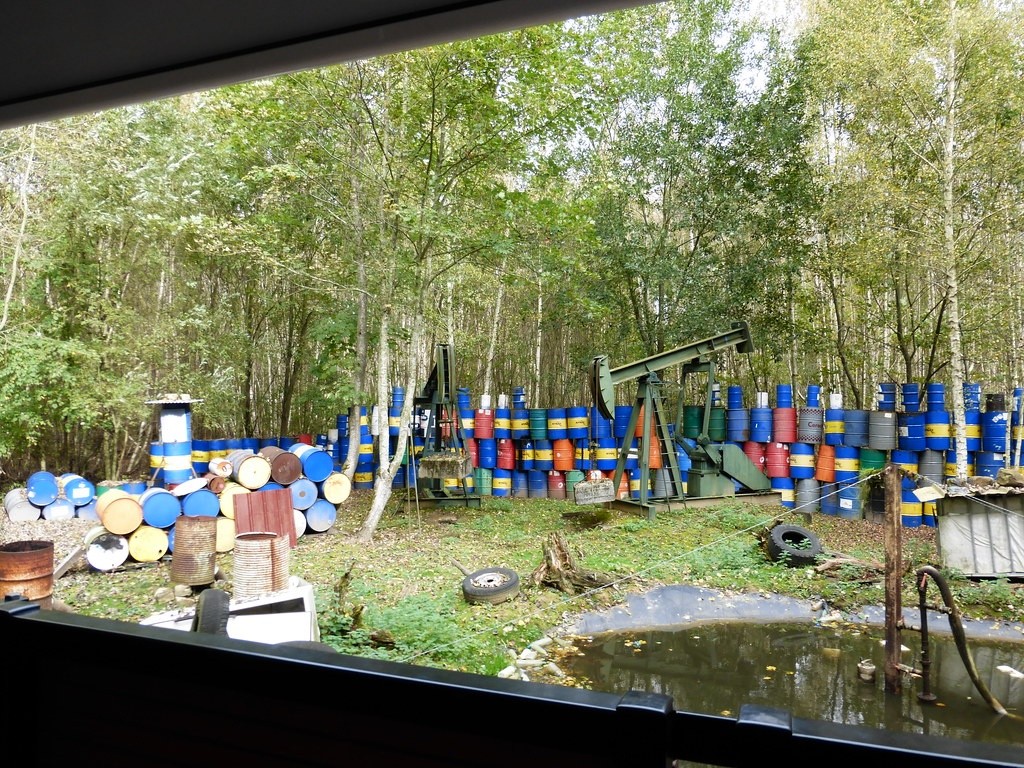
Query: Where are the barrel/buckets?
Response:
[373,385,675,502]
[0,407,373,609]
[678,382,1024,526]
